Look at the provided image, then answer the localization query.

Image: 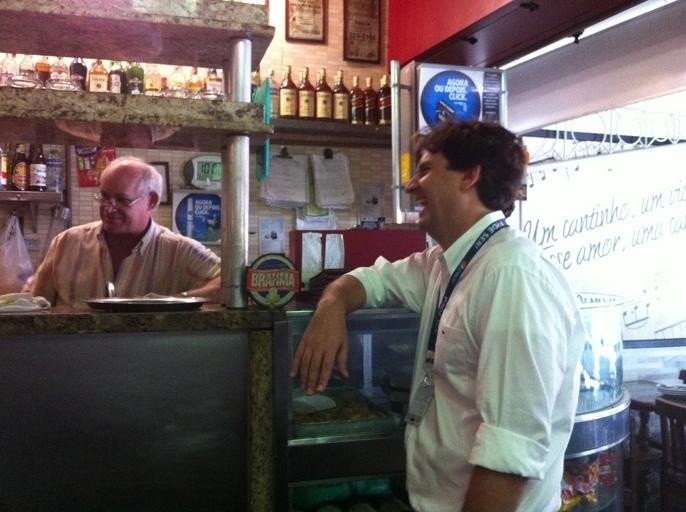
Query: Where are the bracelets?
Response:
[179,291,190,298]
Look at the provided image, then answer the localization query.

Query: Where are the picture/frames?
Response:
[285,0,328,46]
[343,0,382,65]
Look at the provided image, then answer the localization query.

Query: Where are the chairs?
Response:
[650,370,686,450]
[654,398,686,512]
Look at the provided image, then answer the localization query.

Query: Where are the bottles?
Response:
[0,142,47,193]
[250,66,390,126]
[42,205,71,262]
[0,51,222,101]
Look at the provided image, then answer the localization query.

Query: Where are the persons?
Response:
[290,117,582,510]
[22,157,220,304]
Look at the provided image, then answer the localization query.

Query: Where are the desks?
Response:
[622,381,664,511]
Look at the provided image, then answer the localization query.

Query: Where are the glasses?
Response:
[95,192,145,207]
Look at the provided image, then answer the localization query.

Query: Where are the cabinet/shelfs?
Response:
[285,308,632,510]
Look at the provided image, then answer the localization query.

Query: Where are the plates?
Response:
[87,296,213,311]
